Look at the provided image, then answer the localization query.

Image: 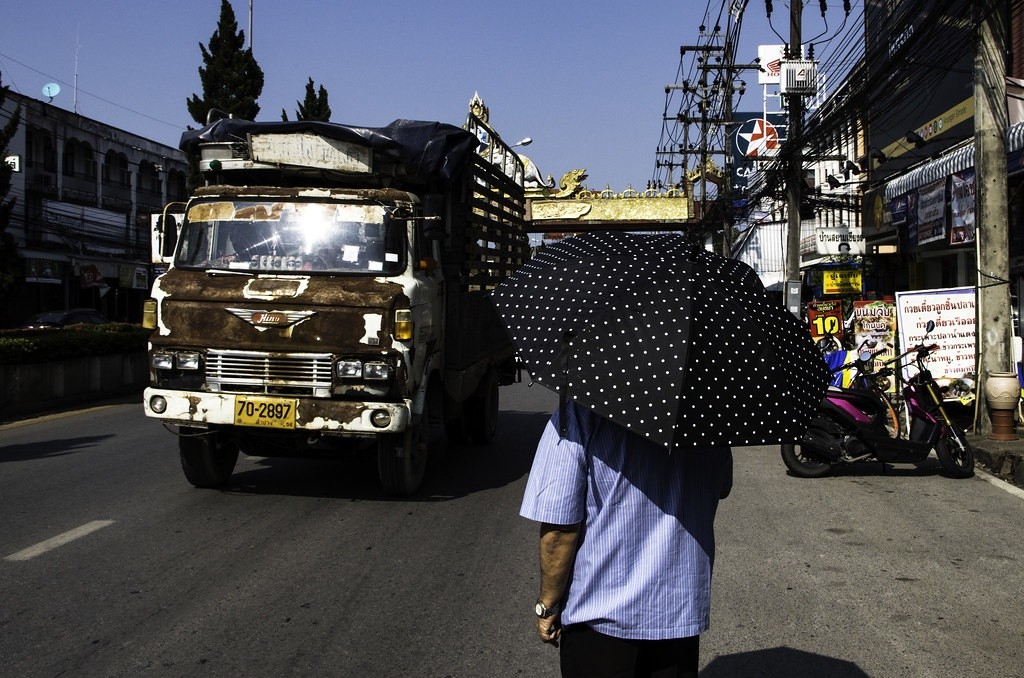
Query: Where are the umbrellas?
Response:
[488,229,830,456]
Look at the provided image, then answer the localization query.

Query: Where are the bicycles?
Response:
[823,346,901,440]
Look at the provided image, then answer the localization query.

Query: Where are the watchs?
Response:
[535,597,560,619]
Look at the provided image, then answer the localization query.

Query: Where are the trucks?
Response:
[142,108,528,497]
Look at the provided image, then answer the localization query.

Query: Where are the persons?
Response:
[518,399,734,674]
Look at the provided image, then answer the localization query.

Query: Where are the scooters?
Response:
[780,321,976,480]
[811,320,847,364]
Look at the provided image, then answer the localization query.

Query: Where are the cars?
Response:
[0,307,112,334]
